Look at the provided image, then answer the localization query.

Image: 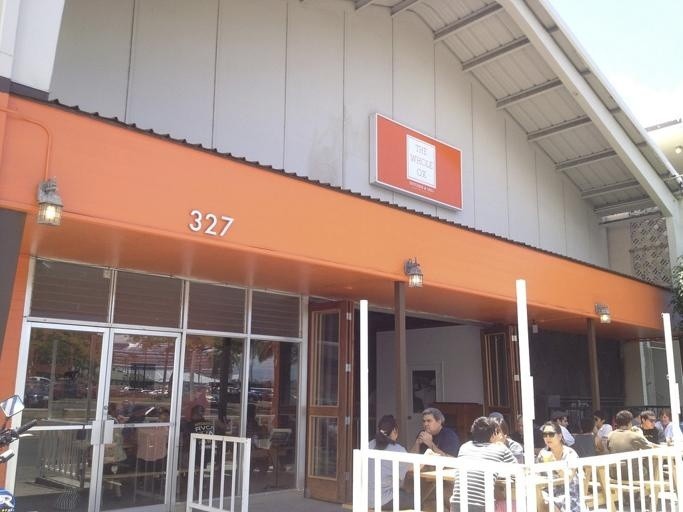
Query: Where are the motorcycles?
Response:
[0,393,38,512]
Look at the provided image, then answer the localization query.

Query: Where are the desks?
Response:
[406,465,560,500]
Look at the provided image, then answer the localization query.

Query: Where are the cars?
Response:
[25,372,295,422]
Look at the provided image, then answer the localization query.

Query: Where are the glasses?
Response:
[647,418,656,423]
[541,432,556,438]
[591,418,597,421]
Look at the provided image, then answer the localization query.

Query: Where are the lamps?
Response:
[595,304,611,325]
[35,177,64,227]
[404,258,424,289]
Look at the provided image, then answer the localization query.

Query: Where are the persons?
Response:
[367,406,682,512]
[100,402,265,485]
